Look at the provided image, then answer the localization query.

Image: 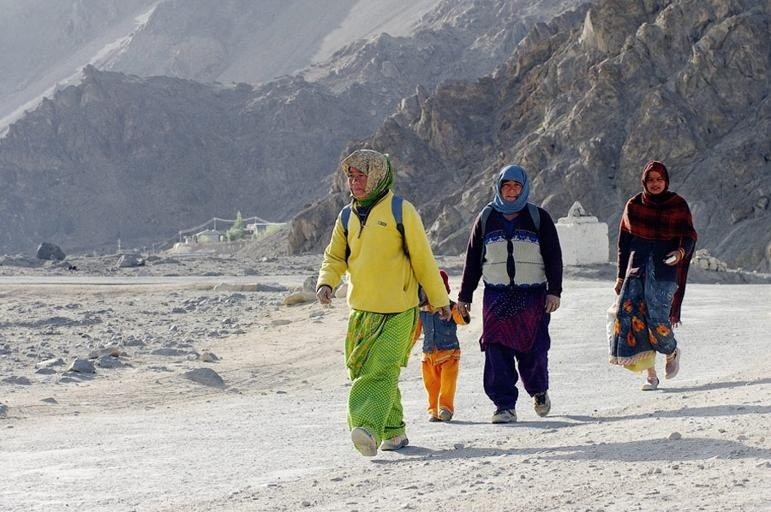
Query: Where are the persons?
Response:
[603,160,698,391]
[312,150,455,457]
[454,164,562,423]
[408,270,471,425]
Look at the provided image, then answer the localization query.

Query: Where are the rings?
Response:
[556,304,559,308]
[552,304,556,308]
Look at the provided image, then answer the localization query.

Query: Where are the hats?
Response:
[440,270,450,294]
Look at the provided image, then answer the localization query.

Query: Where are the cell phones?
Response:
[665,255,676,264]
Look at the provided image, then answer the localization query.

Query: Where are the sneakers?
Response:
[381,433,408,450]
[429,417,437,421]
[665,348,680,379]
[641,375,659,390]
[440,410,451,421]
[533,392,551,417]
[351,427,377,456]
[493,408,517,422]
